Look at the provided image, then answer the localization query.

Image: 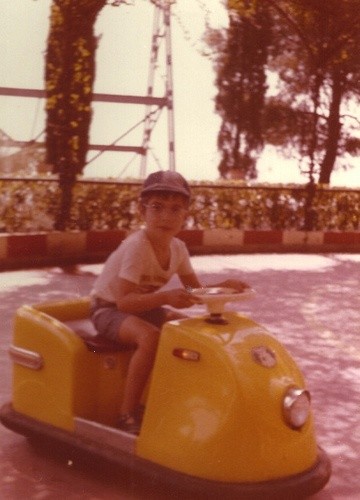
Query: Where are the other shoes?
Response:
[119,413,140,433]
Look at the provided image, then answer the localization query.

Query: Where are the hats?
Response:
[141,171,192,198]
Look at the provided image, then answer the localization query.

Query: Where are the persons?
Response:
[90,171,251,433]
